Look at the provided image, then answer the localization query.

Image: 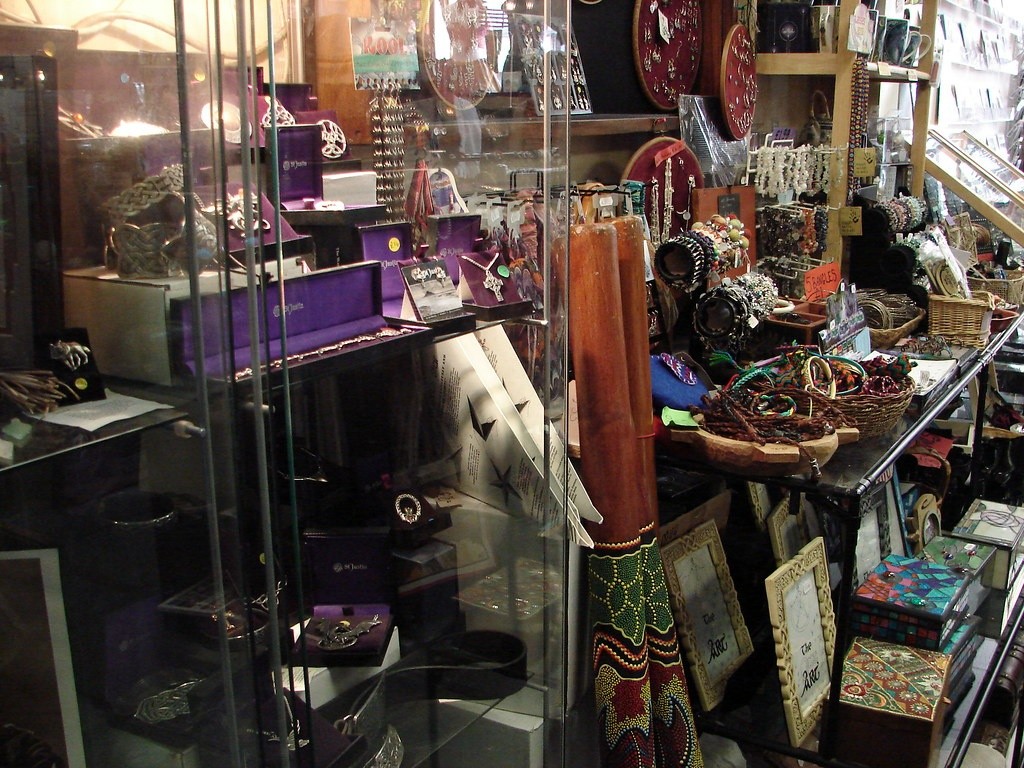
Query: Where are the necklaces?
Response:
[461,253,504,302]
[847,56,870,208]
[650,157,696,332]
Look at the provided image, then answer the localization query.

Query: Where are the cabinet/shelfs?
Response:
[733,0,939,288]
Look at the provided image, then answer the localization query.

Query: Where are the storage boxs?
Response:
[63,73,532,389]
[77,705,200,768]
[816,498,1024,768]
[269,616,402,711]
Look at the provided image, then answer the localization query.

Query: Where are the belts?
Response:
[331,618,529,768]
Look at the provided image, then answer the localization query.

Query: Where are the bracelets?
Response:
[735,36,758,132]
[754,144,843,198]
[642,205,1024,517]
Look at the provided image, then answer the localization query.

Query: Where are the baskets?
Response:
[746,352,916,440]
[927,291,996,347]
[968,269,1024,305]
[869,306,925,350]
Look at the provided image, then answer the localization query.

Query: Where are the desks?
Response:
[652,299,1024,768]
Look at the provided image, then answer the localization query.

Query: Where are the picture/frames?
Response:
[763,537,839,750]
[658,518,755,711]
[742,462,913,613]
[904,492,942,557]
